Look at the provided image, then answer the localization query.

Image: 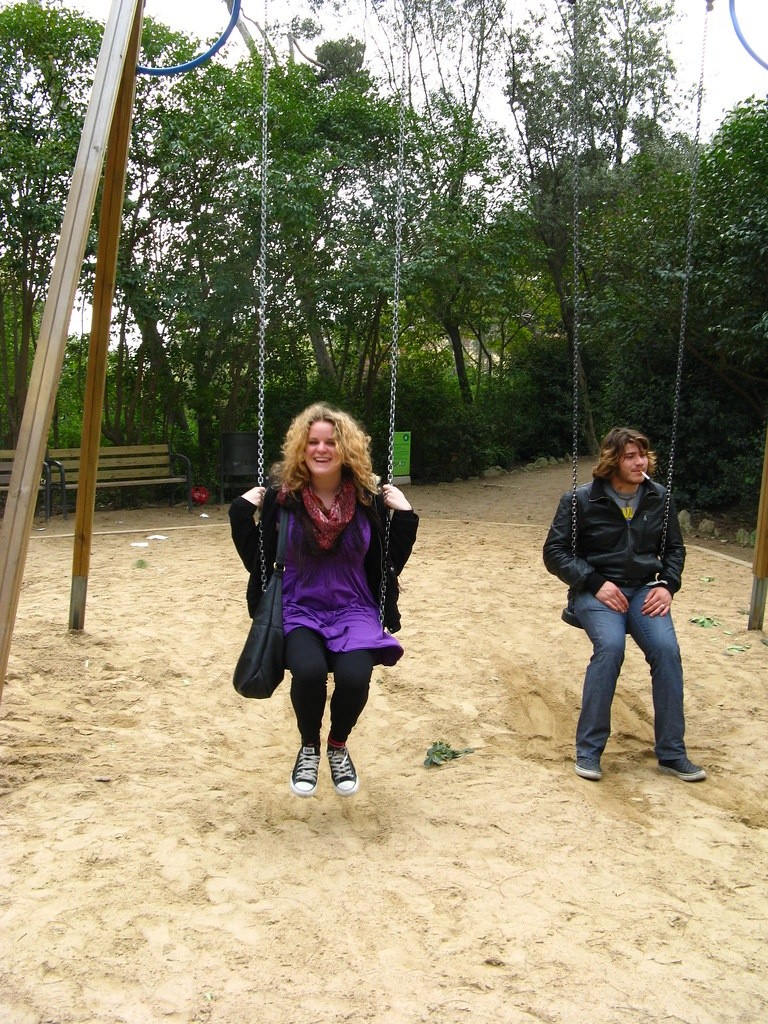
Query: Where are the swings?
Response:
[259,0,405,663]
[559,1,707,630]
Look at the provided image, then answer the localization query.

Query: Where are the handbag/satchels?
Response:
[232,508,289,699]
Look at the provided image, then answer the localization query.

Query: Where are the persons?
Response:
[543,424,705,783]
[227,399,418,797]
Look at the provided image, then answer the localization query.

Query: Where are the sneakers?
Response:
[575,756,603,780]
[289,745,321,797]
[657,759,706,782]
[327,747,359,797]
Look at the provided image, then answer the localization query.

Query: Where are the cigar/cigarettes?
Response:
[639,470,651,480]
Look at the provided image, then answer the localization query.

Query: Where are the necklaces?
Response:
[610,479,642,508]
